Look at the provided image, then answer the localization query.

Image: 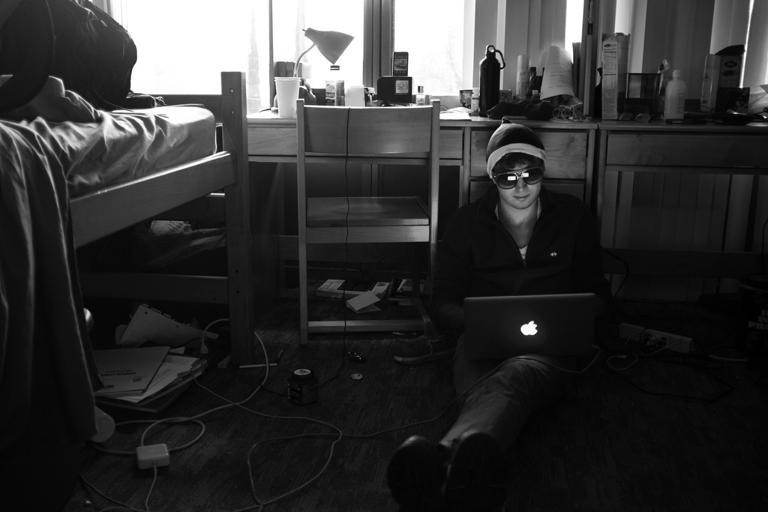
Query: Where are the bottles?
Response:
[515,54,529,98]
[479,44,506,118]
[664,67,687,121]
[335,79,345,105]
[416,85,425,104]
[326,64,343,102]
[470,96,479,116]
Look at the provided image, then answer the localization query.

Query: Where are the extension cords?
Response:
[617,322,697,355]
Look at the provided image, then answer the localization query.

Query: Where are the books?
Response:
[88,302,223,423]
[540,28,631,122]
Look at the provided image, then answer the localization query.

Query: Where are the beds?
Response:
[0,70,253,439]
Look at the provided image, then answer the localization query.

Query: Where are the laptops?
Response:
[463,293,598,369]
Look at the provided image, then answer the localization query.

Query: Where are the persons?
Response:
[385,116,608,508]
[1,0,169,116]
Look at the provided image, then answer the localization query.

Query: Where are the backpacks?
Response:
[0,0,137,112]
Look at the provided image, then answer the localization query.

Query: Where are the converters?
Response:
[135,442,171,472]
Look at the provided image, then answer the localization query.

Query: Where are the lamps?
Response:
[288,28,354,106]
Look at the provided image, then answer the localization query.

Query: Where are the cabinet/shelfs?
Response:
[603,124,767,258]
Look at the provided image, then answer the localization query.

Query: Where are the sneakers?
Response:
[441,428,506,511]
[387,436,447,510]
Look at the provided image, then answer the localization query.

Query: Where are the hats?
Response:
[486,119,546,179]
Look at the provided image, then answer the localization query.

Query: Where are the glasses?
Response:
[493,165,545,189]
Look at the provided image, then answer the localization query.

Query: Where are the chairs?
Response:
[292,101,436,347]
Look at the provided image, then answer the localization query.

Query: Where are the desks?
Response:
[248,106,596,335]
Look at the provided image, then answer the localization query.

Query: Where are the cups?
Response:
[274,76,301,117]
[460,90,474,108]
